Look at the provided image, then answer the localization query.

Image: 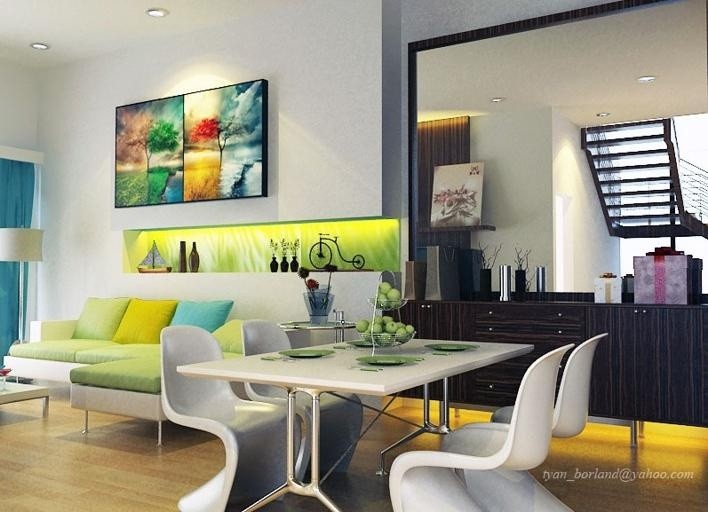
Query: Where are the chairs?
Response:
[490,332,608,512]
[389,342,576,512]
[160,326,310,512]
[243,320,362,478]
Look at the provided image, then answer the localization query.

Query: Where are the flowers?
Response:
[298,264,338,310]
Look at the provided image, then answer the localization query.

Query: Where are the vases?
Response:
[303,292,335,325]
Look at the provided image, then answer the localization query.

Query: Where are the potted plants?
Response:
[270,238,279,272]
[478,242,500,300]
[513,247,533,299]
[290,238,301,273]
[280,238,289,272]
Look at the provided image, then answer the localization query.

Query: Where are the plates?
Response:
[357,356,424,365]
[278,350,335,358]
[346,341,378,347]
[424,343,480,351]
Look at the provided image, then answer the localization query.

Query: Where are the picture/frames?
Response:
[115,95,183,208]
[183,79,269,203]
[430,161,486,227]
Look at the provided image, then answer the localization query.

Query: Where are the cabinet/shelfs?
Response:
[383,302,708,430]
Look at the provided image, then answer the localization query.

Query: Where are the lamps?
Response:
[0,227,44,383]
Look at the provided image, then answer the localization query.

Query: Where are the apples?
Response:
[378,295,389,307]
[355,316,414,347]
[379,282,391,295]
[387,288,402,300]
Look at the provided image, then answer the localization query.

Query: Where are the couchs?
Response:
[2,319,244,446]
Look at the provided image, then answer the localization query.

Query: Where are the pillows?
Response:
[212,320,243,353]
[167,301,234,333]
[72,297,130,340]
[113,298,178,344]
